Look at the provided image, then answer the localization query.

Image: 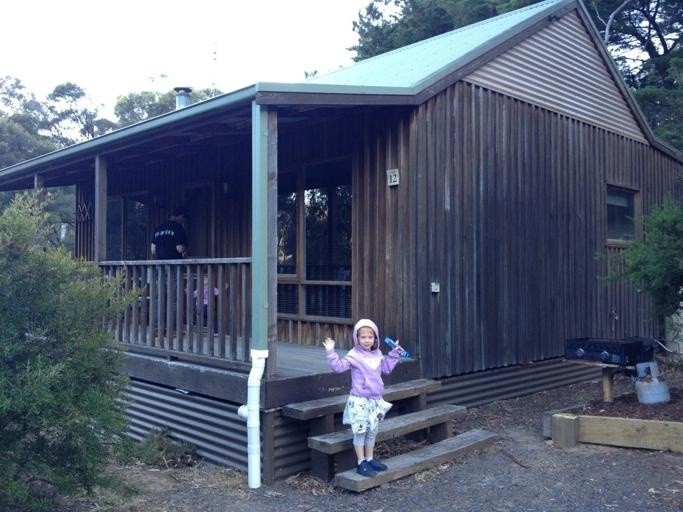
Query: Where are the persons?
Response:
[148,206,189,340]
[322,317,405,479]
[184,274,229,337]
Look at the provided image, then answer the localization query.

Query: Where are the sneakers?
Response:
[356,460,377,478]
[368,457,387,472]
